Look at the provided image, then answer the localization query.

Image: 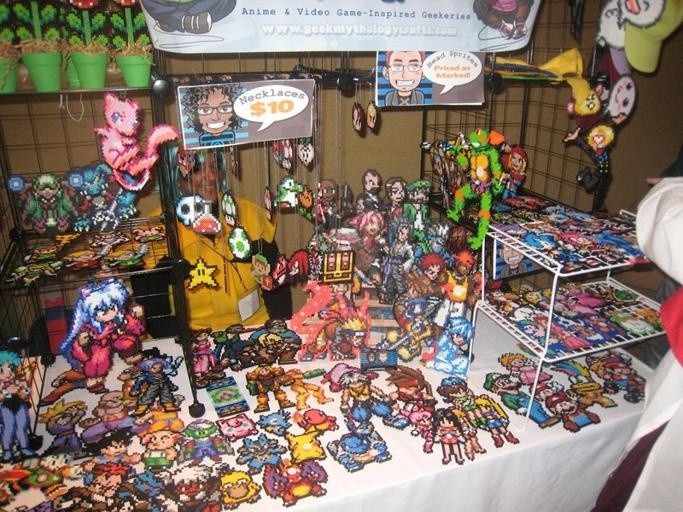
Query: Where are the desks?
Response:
[0,308,655,508]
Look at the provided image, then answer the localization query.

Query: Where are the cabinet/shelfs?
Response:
[465,209,670,431]
[0,74,205,451]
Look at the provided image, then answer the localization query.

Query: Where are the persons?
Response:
[181,83,244,147]
[592,175,683,512]
[129,151,292,340]
[382,51,424,106]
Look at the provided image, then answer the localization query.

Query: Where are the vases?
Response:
[2,52,152,93]
